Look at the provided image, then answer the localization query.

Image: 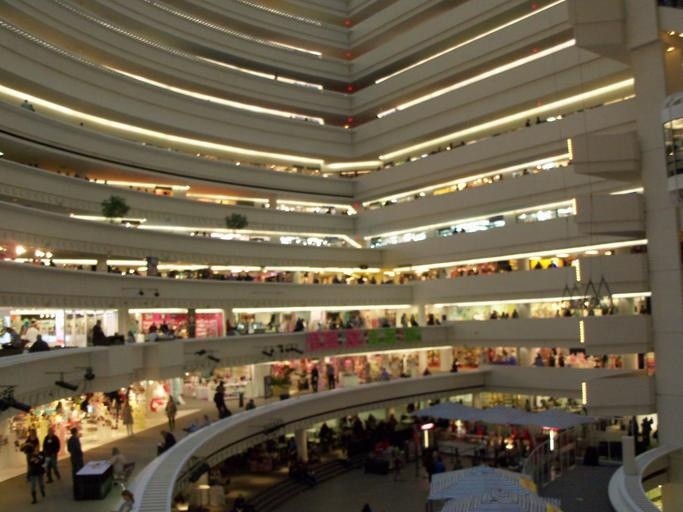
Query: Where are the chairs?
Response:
[109,461,135,491]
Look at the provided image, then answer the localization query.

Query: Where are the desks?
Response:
[75,461,113,500]
[191,484,224,506]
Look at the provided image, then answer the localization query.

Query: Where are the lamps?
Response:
[574,258,587,407]
[47,366,98,390]
[0,384,31,413]
[196,349,220,363]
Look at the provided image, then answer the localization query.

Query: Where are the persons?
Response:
[28,311,446,354]
[213,364,336,417]
[488,301,648,370]
[250,398,657,509]
[19,425,135,509]
[80,390,178,457]
